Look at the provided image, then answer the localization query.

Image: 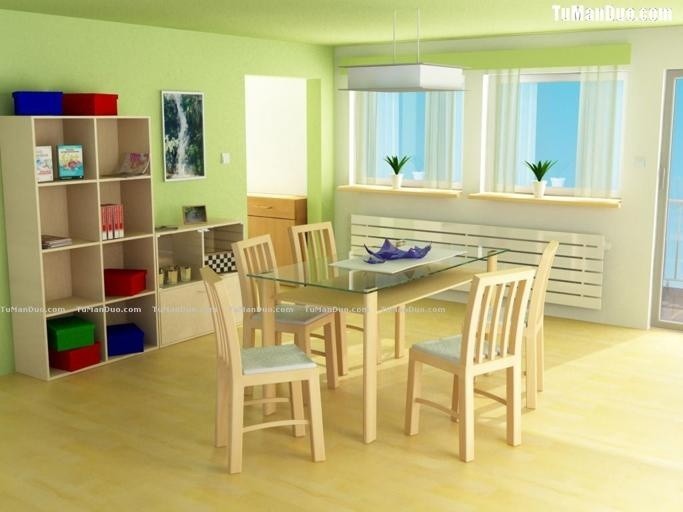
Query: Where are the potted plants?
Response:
[524,159,559,198]
[383,155,410,189]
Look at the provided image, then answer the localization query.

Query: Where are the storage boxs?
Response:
[47,316,96,353]
[12,90,64,116]
[107,323,144,356]
[49,341,102,371]
[62,93,118,116]
[104,268,147,296]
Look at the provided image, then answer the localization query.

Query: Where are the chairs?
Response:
[198,265,325,475]
[287,221,382,376]
[231,234,338,396]
[460,240,560,409]
[404,266,537,463]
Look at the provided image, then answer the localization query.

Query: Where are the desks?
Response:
[245,238,511,443]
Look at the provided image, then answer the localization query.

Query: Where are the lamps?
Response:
[337,8,472,93]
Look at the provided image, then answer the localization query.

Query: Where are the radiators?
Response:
[348,212,604,310]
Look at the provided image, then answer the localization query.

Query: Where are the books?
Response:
[100,203,124,240]
[36,145,55,183]
[56,143,85,180]
[119,151,149,176]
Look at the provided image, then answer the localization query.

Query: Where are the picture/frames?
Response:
[161,89,206,183]
[180,204,209,226]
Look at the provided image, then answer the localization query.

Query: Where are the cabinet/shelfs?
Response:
[154,217,245,349]
[247,193,307,288]
[0,113,158,382]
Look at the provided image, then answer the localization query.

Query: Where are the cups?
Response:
[157,265,190,287]
